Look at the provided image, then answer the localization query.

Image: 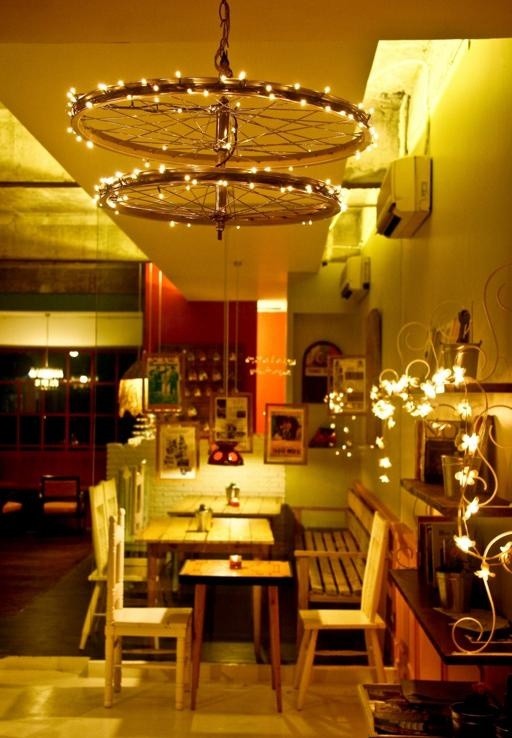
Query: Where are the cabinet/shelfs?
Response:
[389,382,512,668]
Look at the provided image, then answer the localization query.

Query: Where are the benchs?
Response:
[293,487,391,657]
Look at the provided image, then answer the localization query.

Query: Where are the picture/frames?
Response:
[141,352,374,482]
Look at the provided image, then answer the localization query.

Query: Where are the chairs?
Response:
[293,511,389,712]
[40,476,87,542]
[78,458,195,712]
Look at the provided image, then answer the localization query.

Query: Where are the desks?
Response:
[136,494,293,714]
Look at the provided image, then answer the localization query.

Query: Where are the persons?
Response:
[150,365,178,402]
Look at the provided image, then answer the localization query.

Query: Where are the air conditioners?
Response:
[339,255,370,305]
[375,156,432,239]
